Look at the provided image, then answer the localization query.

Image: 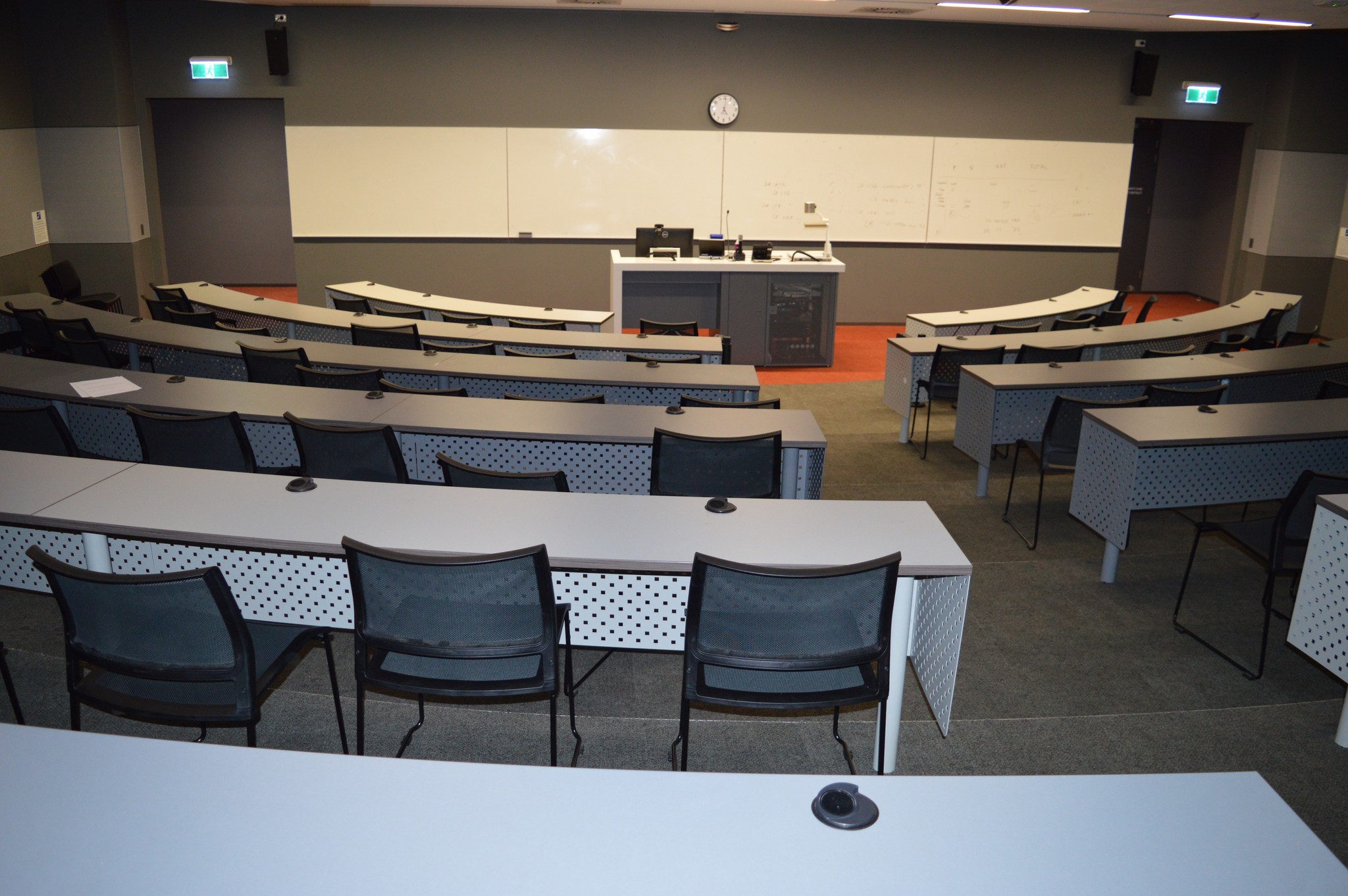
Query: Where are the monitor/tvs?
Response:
[635,227,694,258]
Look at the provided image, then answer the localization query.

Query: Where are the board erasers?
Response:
[518,232,532,237]
[710,234,724,239]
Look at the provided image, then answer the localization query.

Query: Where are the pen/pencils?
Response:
[773,258,781,261]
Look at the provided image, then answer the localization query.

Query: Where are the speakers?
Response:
[1130,50,1159,95]
[265,27,289,76]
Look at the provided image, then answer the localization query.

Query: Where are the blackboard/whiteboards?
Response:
[286,128,1133,251]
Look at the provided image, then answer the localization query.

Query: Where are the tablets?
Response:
[751,245,773,260]
[699,239,725,257]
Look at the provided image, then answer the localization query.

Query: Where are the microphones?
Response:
[726,210,729,252]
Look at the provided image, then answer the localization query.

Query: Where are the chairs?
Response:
[118,398,261,474]
[1016,341,1086,365]
[908,340,1006,462]
[1098,305,1131,327]
[1144,342,1196,359]
[276,405,410,482]
[148,279,194,312]
[1001,390,1152,552]
[1316,378,1348,400]
[234,338,312,386]
[1230,302,1297,351]
[638,313,702,337]
[165,305,217,329]
[39,255,124,314]
[295,362,386,392]
[990,320,1042,336]
[1137,291,1159,322]
[1051,314,1097,331]
[374,305,427,320]
[24,542,350,753]
[1201,332,1252,355]
[348,317,423,352]
[649,423,783,498]
[215,321,272,336]
[440,309,493,326]
[1277,323,1321,348]
[55,328,119,369]
[668,548,907,776]
[1146,373,1231,408]
[1167,466,1348,679]
[379,376,469,397]
[140,293,184,324]
[435,452,571,492]
[343,529,583,765]
[37,310,99,364]
[4,301,50,358]
[328,292,372,314]
[0,403,80,457]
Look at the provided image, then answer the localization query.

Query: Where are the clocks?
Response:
[707,93,740,126]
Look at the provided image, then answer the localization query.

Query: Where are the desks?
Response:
[0,280,1348,896]
[605,246,846,369]
